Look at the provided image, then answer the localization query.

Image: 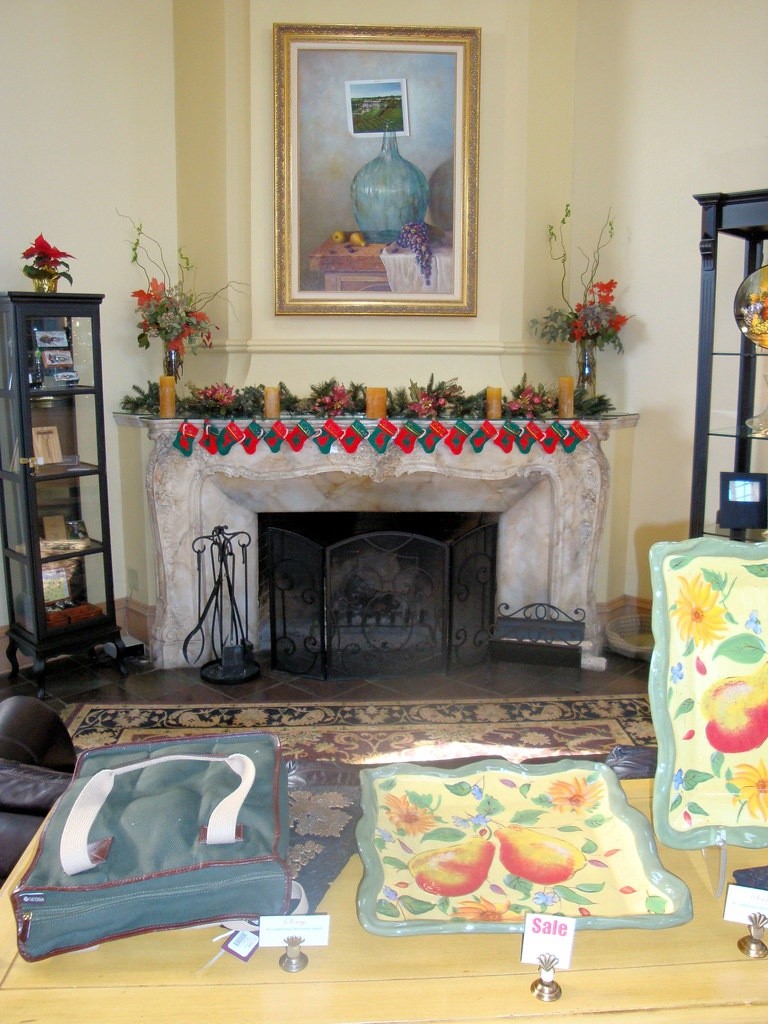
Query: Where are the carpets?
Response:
[61,691,658,764]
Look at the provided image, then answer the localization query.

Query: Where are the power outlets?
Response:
[126,568,140,592]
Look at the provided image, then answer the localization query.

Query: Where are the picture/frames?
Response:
[271,21,481,320]
[41,567,73,606]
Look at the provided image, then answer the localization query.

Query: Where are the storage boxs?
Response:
[43,514,67,542]
[15,611,68,634]
[63,603,103,623]
[122,635,145,658]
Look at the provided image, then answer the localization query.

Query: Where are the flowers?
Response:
[19,232,77,287]
[116,208,250,384]
[120,373,616,420]
[528,202,637,387]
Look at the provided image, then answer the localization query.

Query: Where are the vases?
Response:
[165,349,180,379]
[575,336,598,405]
[32,264,57,293]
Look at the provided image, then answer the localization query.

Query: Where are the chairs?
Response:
[0,695,74,882]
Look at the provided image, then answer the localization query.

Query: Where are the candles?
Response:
[365,387,387,420]
[487,387,502,419]
[558,377,574,419]
[159,376,176,418]
[263,387,281,420]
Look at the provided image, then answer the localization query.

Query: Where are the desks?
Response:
[0,777,768,1024]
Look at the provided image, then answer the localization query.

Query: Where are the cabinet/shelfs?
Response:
[689,189,768,541]
[0,292,131,701]
